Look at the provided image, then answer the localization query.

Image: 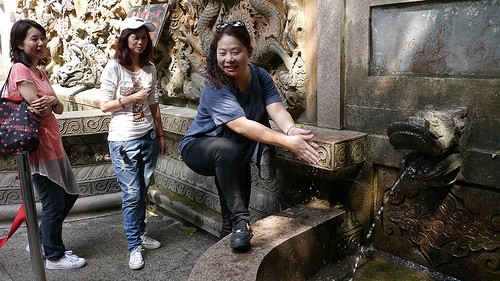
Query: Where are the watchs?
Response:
[52,101,60,108]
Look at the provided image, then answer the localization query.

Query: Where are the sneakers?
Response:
[230,220,251,247]
[26,242,45,255]
[128,245,145,269]
[46,250,86,270]
[141,232,162,250]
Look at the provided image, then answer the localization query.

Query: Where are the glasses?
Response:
[216,20,246,29]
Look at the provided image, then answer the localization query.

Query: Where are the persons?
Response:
[100,16,170,269]
[7,19,87,271]
[179,21,321,248]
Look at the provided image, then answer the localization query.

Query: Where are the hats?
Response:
[121,16,156,32]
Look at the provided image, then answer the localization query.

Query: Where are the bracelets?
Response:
[286,126,297,136]
[159,131,165,137]
[118,97,125,109]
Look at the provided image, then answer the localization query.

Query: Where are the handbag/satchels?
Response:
[0,95,40,155]
